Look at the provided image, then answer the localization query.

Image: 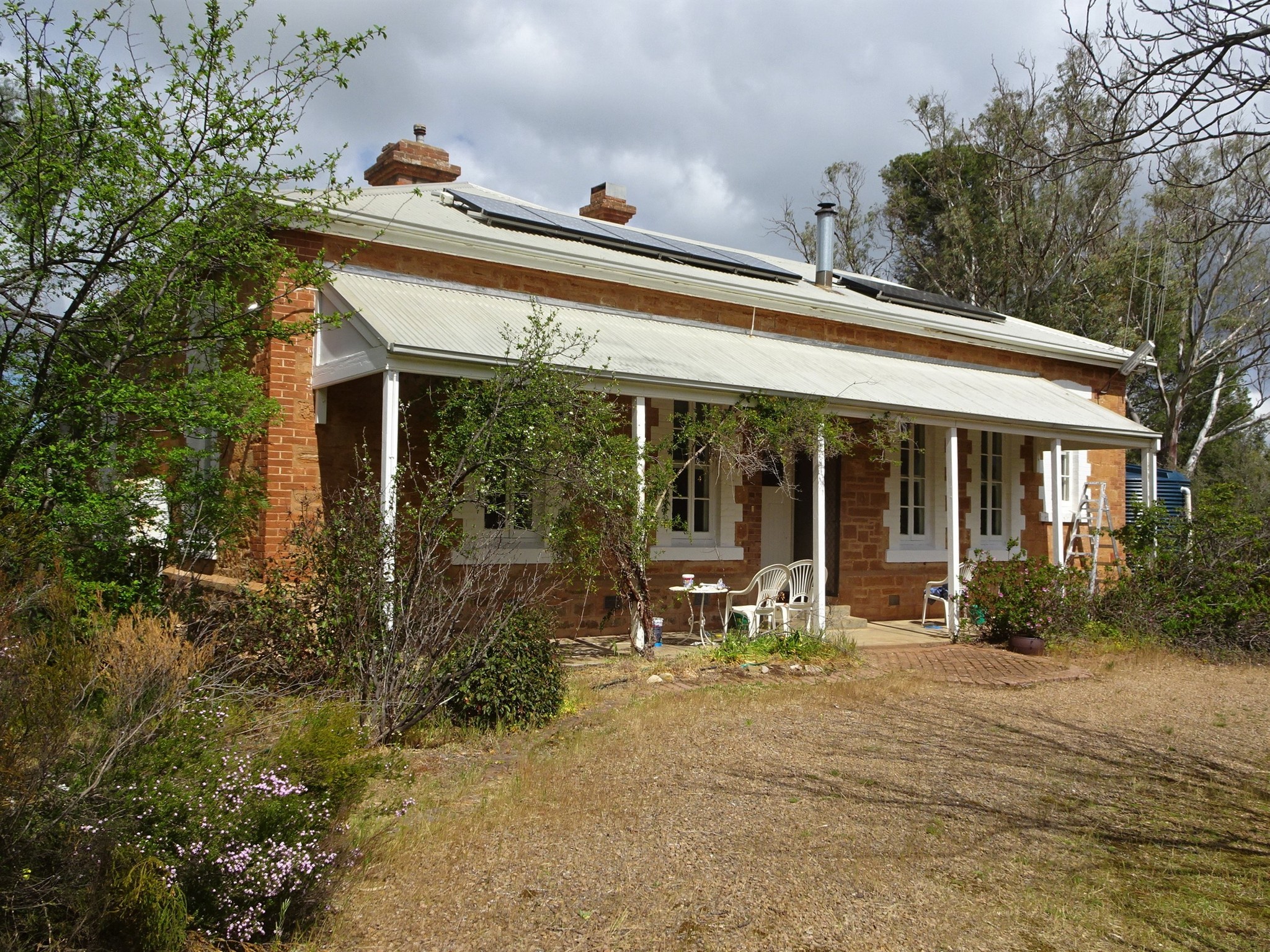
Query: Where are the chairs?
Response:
[921,560,980,636]
[722,563,791,646]
[754,558,828,638]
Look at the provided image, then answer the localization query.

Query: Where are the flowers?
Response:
[949,536,1088,640]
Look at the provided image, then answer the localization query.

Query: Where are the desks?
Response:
[669,586,728,650]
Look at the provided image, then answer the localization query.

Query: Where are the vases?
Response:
[1008,631,1046,657]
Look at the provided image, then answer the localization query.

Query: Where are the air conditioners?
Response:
[113,475,170,550]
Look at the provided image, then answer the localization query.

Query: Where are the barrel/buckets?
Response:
[682,573,695,589]
[654,617,663,644]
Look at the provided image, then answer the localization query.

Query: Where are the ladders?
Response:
[1060,481,1124,600]
